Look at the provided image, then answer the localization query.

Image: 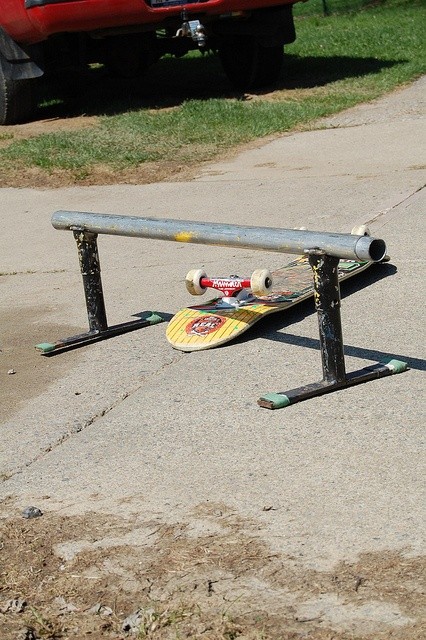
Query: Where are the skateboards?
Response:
[165,225,390,353]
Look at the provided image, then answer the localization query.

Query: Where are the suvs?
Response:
[0,0,297,125]
